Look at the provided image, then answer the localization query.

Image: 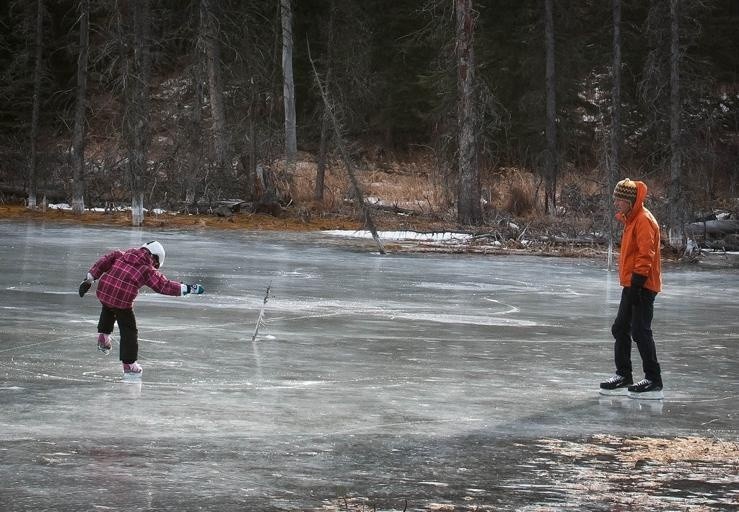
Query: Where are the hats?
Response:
[614,178,638,210]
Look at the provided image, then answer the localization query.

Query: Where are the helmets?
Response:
[139,239,167,270]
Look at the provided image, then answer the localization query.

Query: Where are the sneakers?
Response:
[599,374,634,390]
[96,332,113,351]
[123,361,144,374]
[628,378,664,393]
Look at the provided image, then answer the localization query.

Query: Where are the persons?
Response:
[77,239,206,375]
[597,176,664,393]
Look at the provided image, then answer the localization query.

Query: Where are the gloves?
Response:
[78,271,95,298]
[180,282,205,296]
[628,271,648,305]
